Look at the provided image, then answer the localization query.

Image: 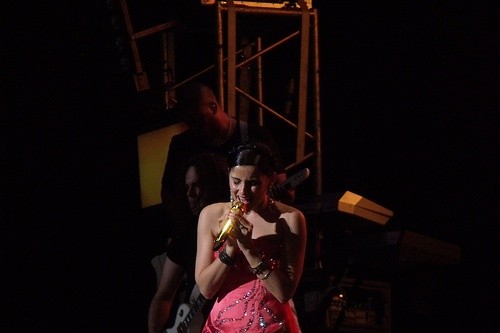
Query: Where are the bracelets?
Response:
[218,247,235,267]
[248,258,274,280]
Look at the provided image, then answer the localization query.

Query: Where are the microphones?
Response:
[213,202,246,252]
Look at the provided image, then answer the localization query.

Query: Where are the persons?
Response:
[194,141,308,333]
[149,83,296,333]
